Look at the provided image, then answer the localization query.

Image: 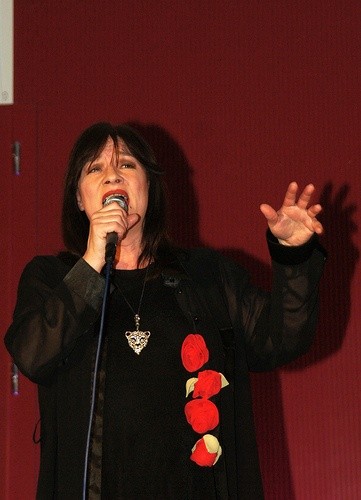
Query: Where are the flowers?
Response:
[180,333,230,468]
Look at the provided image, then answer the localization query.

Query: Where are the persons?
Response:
[1,119,326,499]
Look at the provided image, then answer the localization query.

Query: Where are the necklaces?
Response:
[103,251,153,357]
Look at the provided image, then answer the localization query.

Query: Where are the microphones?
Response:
[104,194,128,261]
